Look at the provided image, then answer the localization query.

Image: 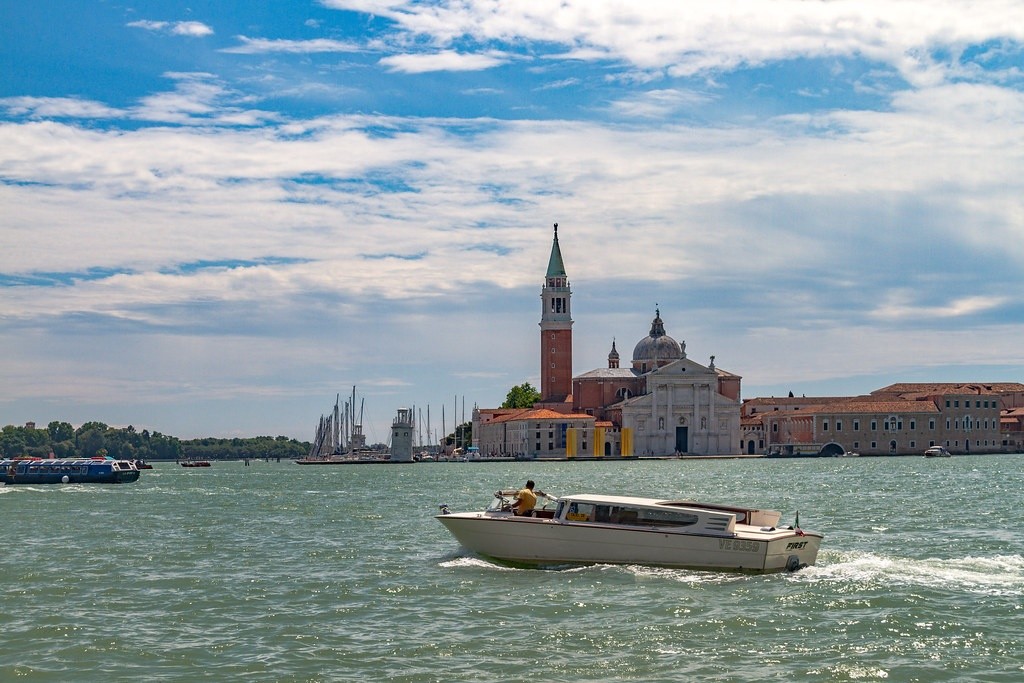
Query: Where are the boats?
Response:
[0,457,140,485]
[923,445,951,457]
[433,478,824,574]
[181,460,211,467]
[767,443,822,457]
[129,457,152,469]
[842,451,860,457]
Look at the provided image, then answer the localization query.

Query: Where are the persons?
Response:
[479,451,521,457]
[503,479,537,516]
[768,446,773,454]
[565,504,589,522]
[776,446,782,452]
